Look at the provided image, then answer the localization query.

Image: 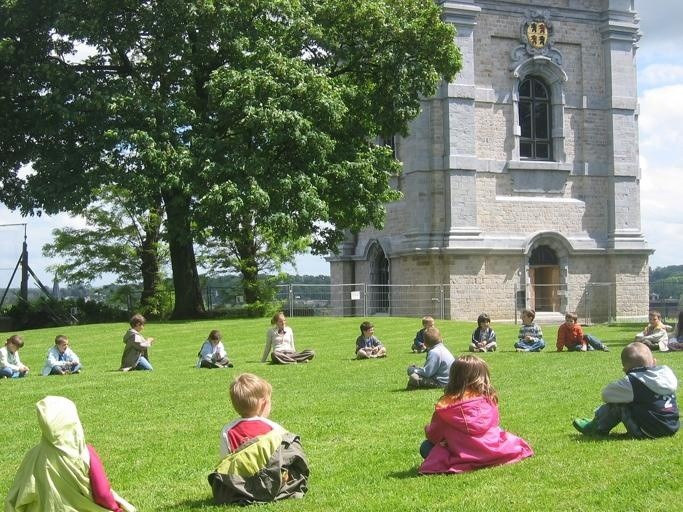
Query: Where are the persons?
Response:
[0,335,29,379]
[9,395,135,512]
[119,314,155,371]
[411,316,443,354]
[574,342,681,440]
[260,312,314,365]
[197,330,233,369]
[355,322,386,360]
[407,327,455,390]
[469,313,497,352]
[418,358,533,475]
[667,312,683,351]
[207,373,310,504]
[556,311,609,352]
[43,336,81,376]
[515,309,545,352]
[634,310,669,352]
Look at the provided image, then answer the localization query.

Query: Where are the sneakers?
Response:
[603,346,609,352]
[573,418,591,436]
